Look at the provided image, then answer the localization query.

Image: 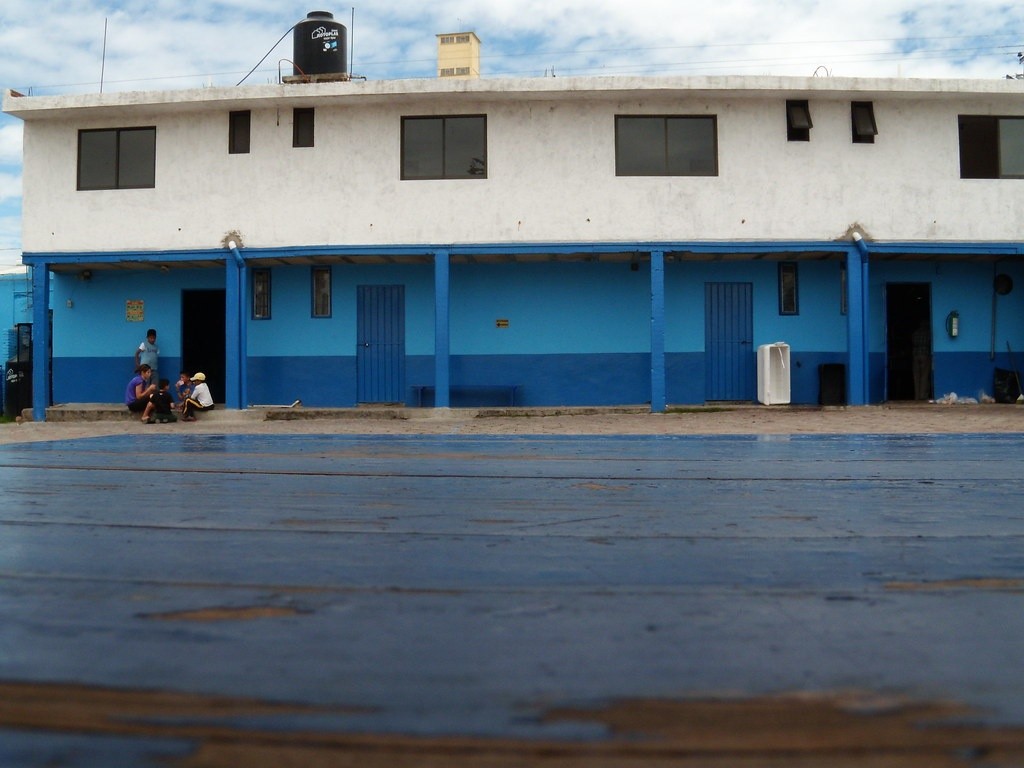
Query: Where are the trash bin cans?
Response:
[993,367,1019,403]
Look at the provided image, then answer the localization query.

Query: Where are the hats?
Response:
[190,373,206,381]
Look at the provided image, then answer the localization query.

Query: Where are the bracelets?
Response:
[176,391,179,393]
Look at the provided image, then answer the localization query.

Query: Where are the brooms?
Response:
[1007,341,1024,405]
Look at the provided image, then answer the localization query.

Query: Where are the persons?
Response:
[914,318,931,400]
[135,329,160,393]
[125,364,157,423]
[181,372,214,421]
[141,378,177,424]
[175,372,197,419]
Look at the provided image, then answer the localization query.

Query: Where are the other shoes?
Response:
[141,417,150,423]
[163,419,168,422]
[182,415,195,421]
[155,419,160,422]
